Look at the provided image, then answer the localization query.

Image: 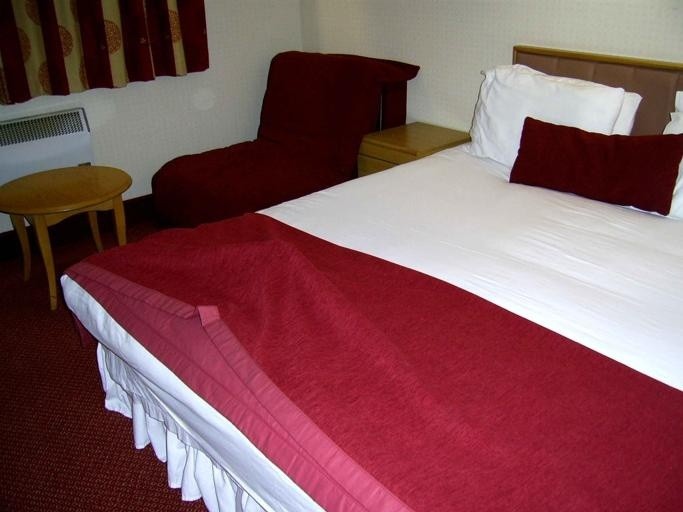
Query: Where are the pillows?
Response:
[467,63,683,221]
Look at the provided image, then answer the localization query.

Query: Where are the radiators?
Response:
[0,108,94,234]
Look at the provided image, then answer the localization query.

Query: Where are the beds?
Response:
[59,45,683,511]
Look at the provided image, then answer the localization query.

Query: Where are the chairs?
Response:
[151,51,420,224]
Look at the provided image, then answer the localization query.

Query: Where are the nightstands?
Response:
[357,122,471,176]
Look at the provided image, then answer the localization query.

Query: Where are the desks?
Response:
[0,166,133,311]
